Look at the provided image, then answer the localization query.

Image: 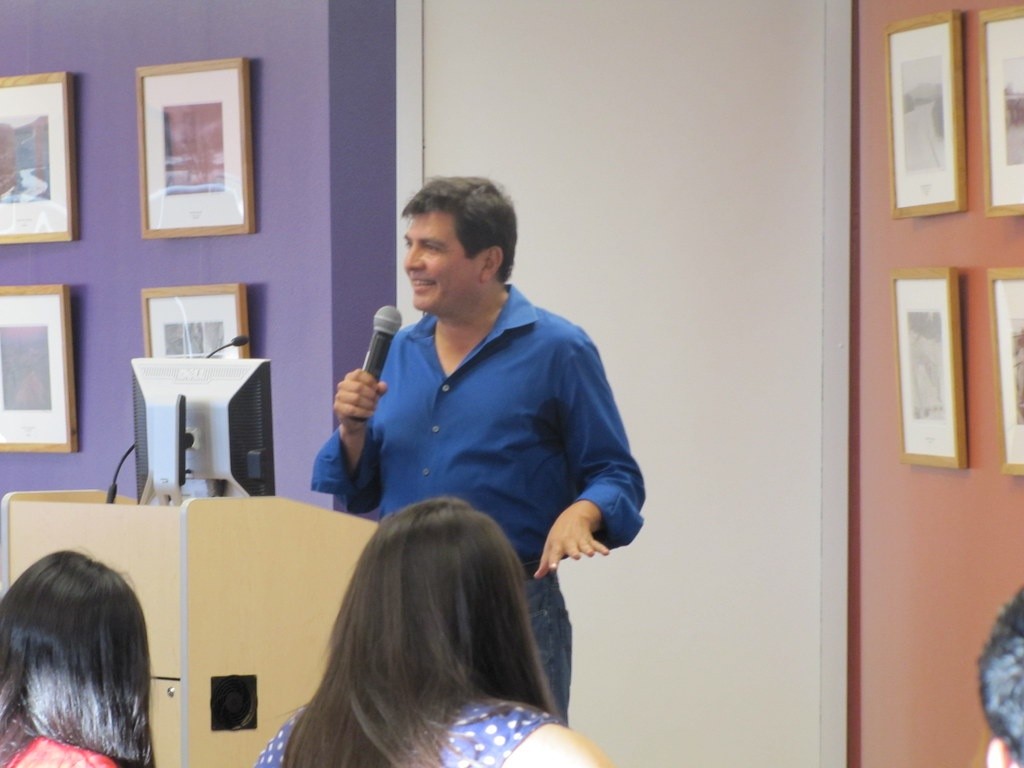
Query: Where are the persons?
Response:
[253,497,609,768]
[976,587,1024,768]
[318,177,649,727]
[0,551,162,768]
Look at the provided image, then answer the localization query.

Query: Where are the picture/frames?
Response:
[986,267,1024,477]
[134,56,258,240]
[979,6,1024,217]
[884,10,968,220]
[889,266,968,470]
[141,282,251,361]
[0,70,80,246]
[0,284,79,454]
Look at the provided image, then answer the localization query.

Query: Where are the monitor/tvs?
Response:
[130,358,276,502]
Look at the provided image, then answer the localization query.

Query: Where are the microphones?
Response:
[349,305,402,421]
[106,336,251,505]
